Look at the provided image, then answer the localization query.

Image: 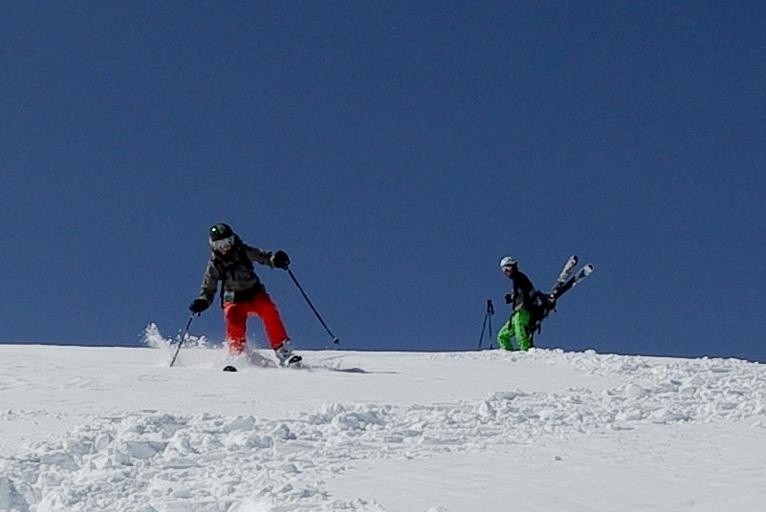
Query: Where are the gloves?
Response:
[189,298,209,313]
[273,250,291,269]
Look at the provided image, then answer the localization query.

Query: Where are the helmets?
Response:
[499,257,517,267]
[208,223,233,241]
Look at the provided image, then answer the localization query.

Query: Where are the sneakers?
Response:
[273,343,302,364]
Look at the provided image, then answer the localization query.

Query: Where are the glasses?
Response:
[501,266,514,273]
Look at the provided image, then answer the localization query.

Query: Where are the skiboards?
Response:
[530,255,594,344]
[222,361,364,372]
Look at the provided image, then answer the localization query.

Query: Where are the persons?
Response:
[493,255,538,351]
[188,222,302,363]
